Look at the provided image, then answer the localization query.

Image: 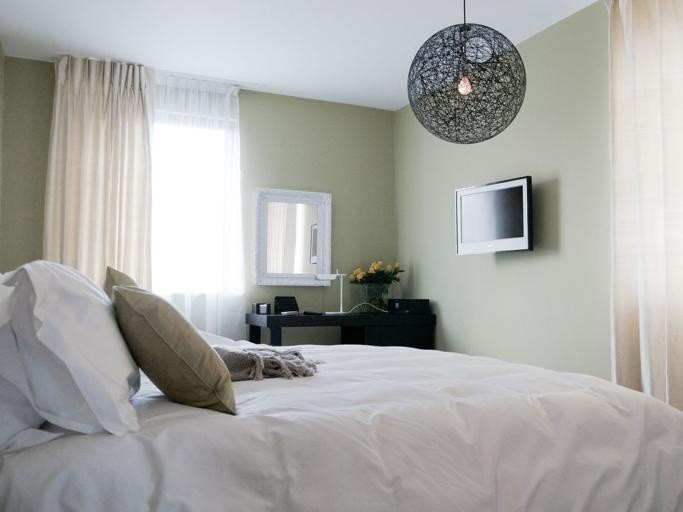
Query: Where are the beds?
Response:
[0,340,683,512]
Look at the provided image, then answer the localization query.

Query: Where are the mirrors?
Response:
[256,187,332,287]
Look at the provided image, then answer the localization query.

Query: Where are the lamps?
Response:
[408,0,526,144]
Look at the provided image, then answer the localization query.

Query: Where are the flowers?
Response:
[348,256,405,289]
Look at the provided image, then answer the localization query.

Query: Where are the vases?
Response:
[358,284,388,312]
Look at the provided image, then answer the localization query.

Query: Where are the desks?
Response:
[246,313,436,351]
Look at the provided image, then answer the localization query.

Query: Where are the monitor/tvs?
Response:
[452,174,534,257]
[310,224,316,264]
